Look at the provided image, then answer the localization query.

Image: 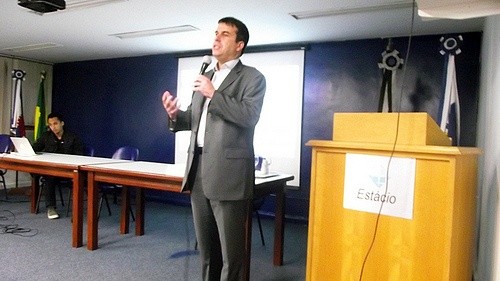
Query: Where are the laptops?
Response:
[10,137,48,156]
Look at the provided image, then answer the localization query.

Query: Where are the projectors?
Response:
[17,0,66,13]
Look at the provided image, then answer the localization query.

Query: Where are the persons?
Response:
[32,112,79,219]
[161,16,267,281]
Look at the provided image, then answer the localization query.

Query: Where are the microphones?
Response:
[195,55,212,86]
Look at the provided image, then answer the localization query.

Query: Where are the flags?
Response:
[378,68,393,113]
[32,70,47,145]
[9,78,29,152]
[439,52,461,147]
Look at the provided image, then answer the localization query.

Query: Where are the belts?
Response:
[195,146,204,154]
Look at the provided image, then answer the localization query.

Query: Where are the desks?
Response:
[0,151,135,248]
[80,160,294,266]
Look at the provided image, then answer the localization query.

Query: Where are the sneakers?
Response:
[48,208,59,220]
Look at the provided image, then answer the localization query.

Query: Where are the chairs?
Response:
[193,157,266,250]
[99,146,140,221]
[34,145,97,216]
[0,134,15,202]
